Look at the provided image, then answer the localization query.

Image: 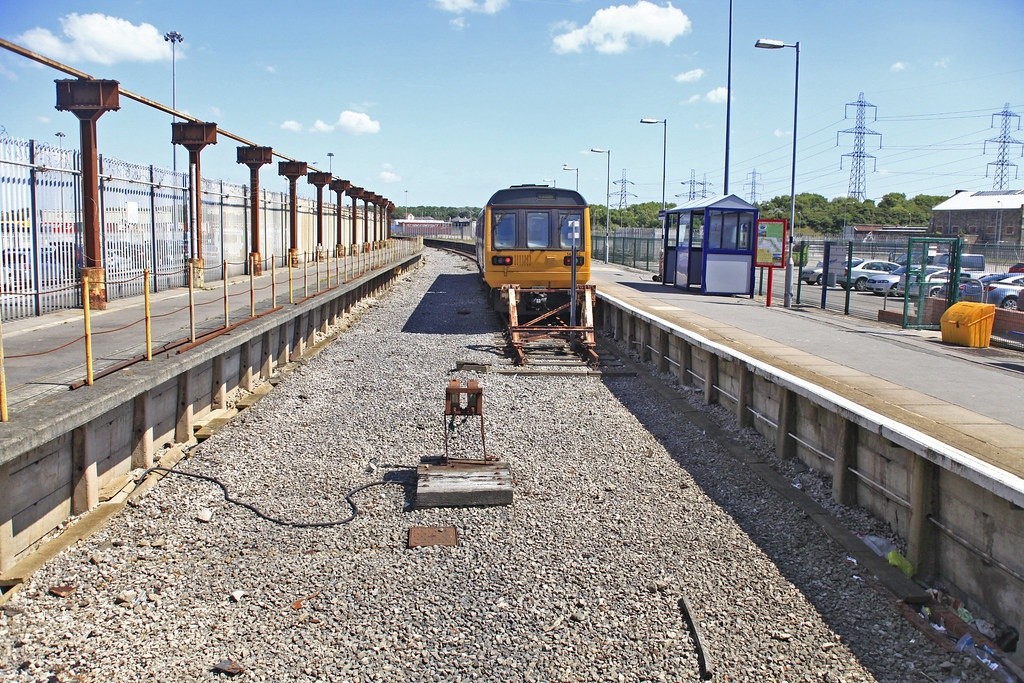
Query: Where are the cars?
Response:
[802,256,1024,311]
[895,253,932,265]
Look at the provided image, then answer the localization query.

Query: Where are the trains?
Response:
[476,185,591,302]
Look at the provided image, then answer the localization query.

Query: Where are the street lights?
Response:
[563,167,578,192]
[754,39,799,309]
[908,212,911,226]
[591,148,610,264]
[327,153,334,203]
[842,201,855,244]
[404,190,408,220]
[164,31,185,171]
[640,118,666,275]
[55,131,66,219]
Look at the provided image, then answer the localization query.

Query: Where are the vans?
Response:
[935,254,985,272]
